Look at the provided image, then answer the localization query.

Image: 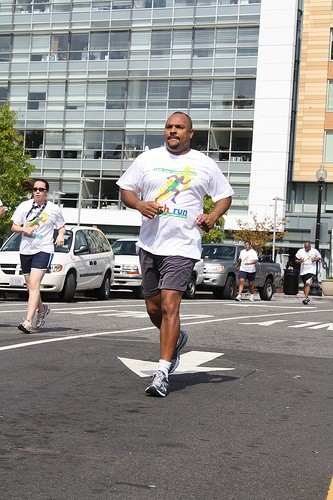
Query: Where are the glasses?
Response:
[33,188,47,192]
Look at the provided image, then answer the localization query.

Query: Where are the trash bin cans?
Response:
[283,269,299,295]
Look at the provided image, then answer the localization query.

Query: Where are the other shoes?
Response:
[235,296,241,300]
[249,297,254,301]
[303,298,310,304]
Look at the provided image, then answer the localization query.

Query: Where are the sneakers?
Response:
[18,322,33,335]
[168,331,189,373]
[36,304,50,327]
[146,370,169,397]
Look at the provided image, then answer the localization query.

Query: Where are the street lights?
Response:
[78,177,95,226]
[272,197,286,262]
[53,191,66,207]
[308,163,327,295]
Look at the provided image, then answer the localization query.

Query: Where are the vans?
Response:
[110,239,204,298]
[0,225,115,302]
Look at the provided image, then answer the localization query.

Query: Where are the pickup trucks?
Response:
[201,244,281,301]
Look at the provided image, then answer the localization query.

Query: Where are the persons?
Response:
[235,241,259,303]
[294,242,322,305]
[116,111,234,397]
[0,179,66,334]
